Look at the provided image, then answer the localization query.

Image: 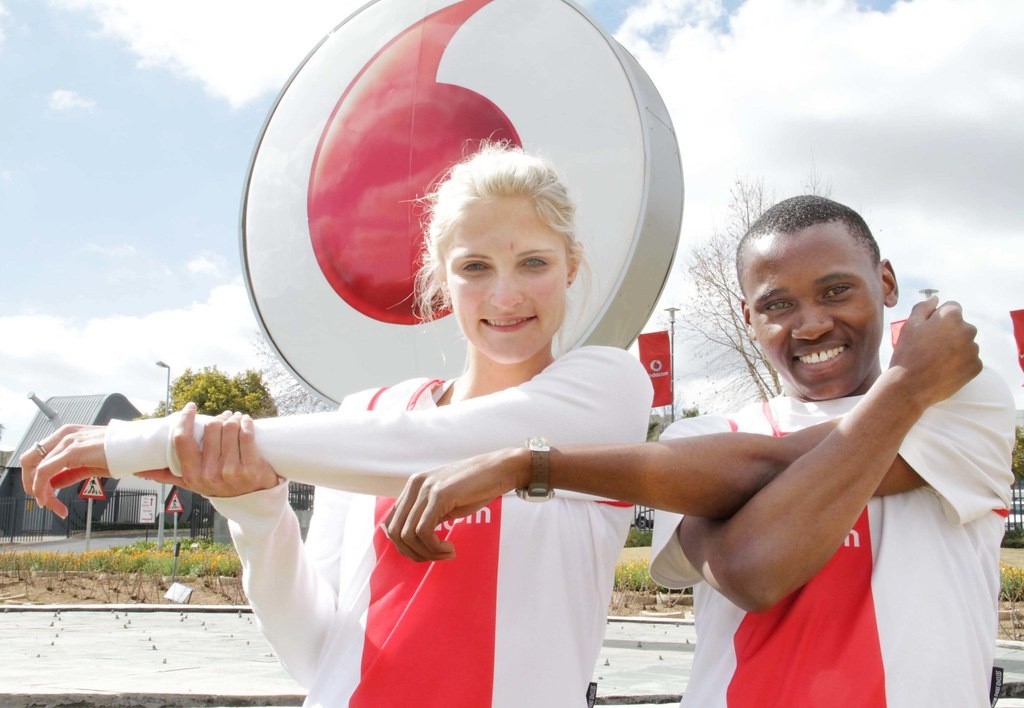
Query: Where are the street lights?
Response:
[155,361,170,554]
[664,306,680,423]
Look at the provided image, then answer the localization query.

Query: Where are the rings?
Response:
[35,442,47,456]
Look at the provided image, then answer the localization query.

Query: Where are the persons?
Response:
[19,141,656,708]
[383,195,1018,708]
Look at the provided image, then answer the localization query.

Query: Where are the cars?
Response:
[1006,501,1023,527]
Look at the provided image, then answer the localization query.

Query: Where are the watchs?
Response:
[515,437,555,503]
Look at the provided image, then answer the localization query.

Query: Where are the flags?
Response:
[1010,310,1024,372]
[637,330,673,408]
